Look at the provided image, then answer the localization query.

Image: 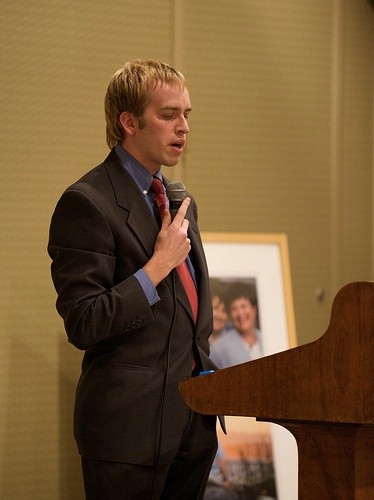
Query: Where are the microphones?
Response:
[166,181,186,222]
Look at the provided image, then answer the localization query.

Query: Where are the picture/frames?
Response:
[200,230,302,499]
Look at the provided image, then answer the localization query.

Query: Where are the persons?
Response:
[215,280,263,367]
[207,278,227,369]
[46,59,226,500]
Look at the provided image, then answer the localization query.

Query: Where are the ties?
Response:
[147,179,198,323]
